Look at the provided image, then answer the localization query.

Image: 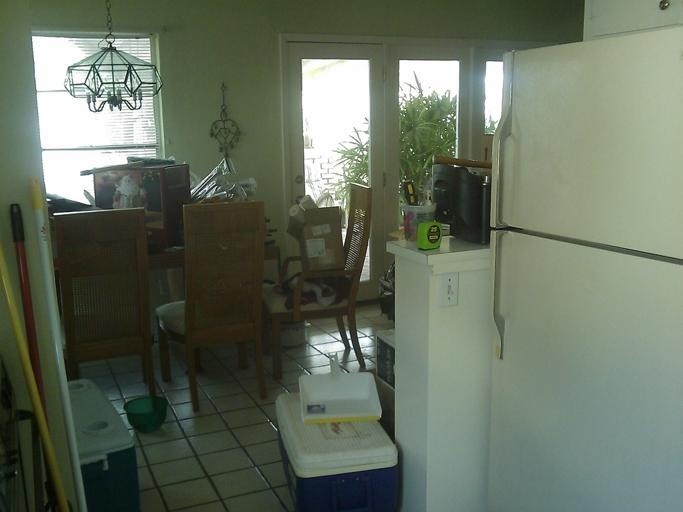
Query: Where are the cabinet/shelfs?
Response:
[92,164,190,252]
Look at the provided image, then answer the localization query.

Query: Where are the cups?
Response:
[401,204,437,251]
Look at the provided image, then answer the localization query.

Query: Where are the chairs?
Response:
[156,201,272,414]
[49,207,165,417]
[262,181,373,378]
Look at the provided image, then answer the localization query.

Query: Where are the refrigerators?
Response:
[483,21,683,512]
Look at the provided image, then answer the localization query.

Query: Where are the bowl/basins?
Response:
[124,396,170,434]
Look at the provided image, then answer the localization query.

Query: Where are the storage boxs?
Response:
[276,393,401,511]
[66,378,140,511]
[368,328,396,436]
[286,206,346,272]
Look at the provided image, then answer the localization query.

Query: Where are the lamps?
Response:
[62,1,164,112]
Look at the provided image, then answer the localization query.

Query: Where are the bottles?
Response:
[416,212,443,251]
